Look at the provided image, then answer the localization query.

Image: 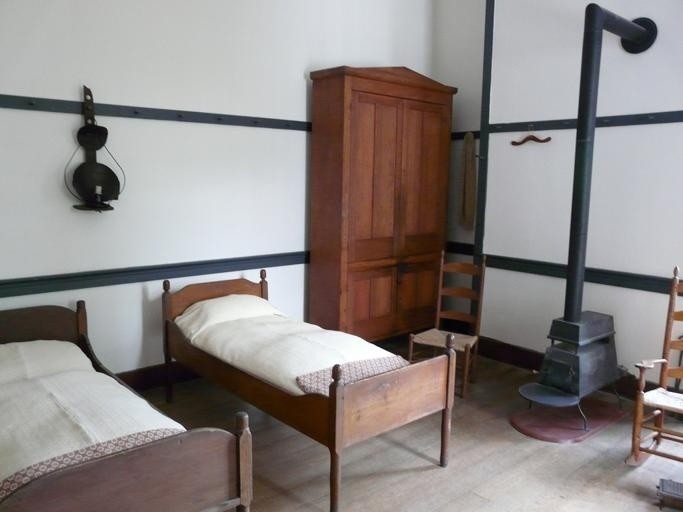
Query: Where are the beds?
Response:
[0,300,254,512]
[161,269,457,512]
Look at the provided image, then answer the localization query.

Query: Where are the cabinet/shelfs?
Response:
[308,66,458,344]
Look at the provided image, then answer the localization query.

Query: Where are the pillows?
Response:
[0,339,93,384]
[183,293,276,325]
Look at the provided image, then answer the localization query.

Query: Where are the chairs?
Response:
[408,248,487,398]
[623,265,683,467]
